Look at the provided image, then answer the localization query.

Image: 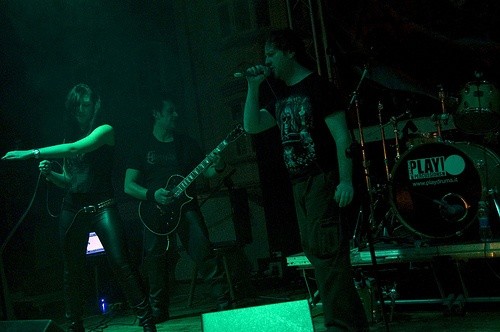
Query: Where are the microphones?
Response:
[448,205,464,214]
[347,64,372,112]
[234,71,264,79]
[381,110,412,129]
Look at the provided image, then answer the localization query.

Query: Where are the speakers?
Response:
[200,298,316,332]
[174,196,252,283]
[0,319,66,332]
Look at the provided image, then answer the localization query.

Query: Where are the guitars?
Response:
[138,124,252,236]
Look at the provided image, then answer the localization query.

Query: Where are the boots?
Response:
[129,293,156,332]
[64,296,85,332]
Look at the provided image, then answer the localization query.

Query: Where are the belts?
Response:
[65,197,115,214]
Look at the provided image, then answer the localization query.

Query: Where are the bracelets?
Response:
[215,167,225,172]
[146,186,160,202]
[34,150,40,159]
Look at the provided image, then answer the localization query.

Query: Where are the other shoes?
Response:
[140,310,169,325]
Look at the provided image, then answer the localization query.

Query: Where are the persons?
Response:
[121,91,233,323]
[0,83,157,332]
[242,28,369,332]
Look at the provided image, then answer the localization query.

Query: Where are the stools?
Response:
[189,240,238,305]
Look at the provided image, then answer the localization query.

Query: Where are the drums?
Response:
[388,138,500,241]
[452,79,500,134]
